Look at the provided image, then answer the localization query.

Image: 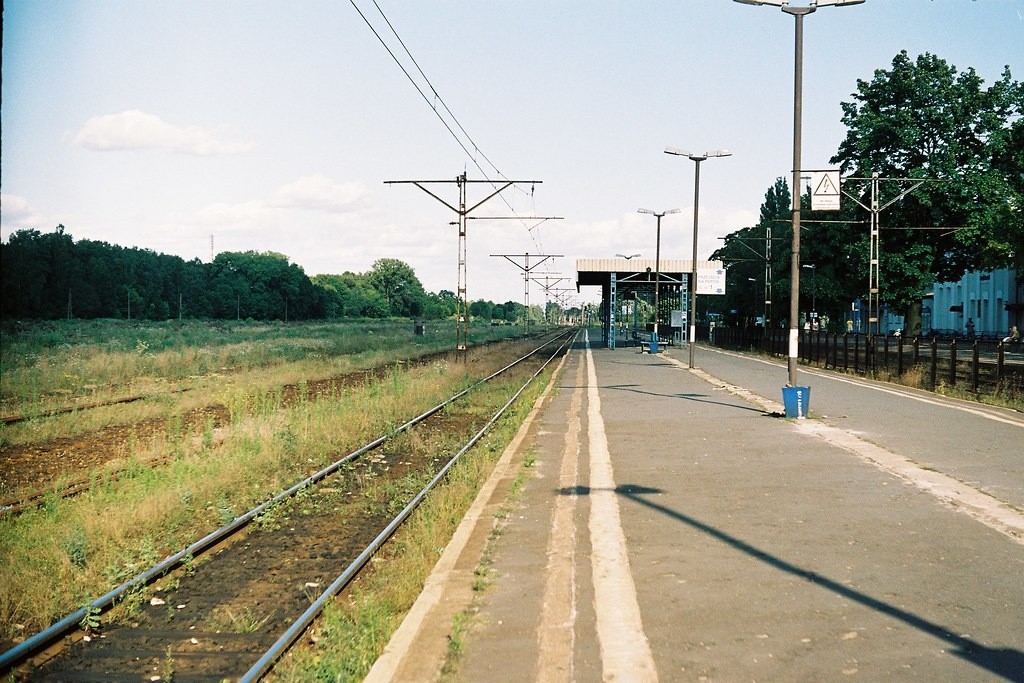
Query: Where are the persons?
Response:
[893,328,901,339]
[1003,326,1020,353]
[804,318,861,335]
[965,318,975,338]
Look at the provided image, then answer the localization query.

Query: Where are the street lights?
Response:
[733,0,867,385]
[637,207,681,333]
[803,264,816,311]
[616,253,642,339]
[663,145,733,368]
[748,278,758,311]
